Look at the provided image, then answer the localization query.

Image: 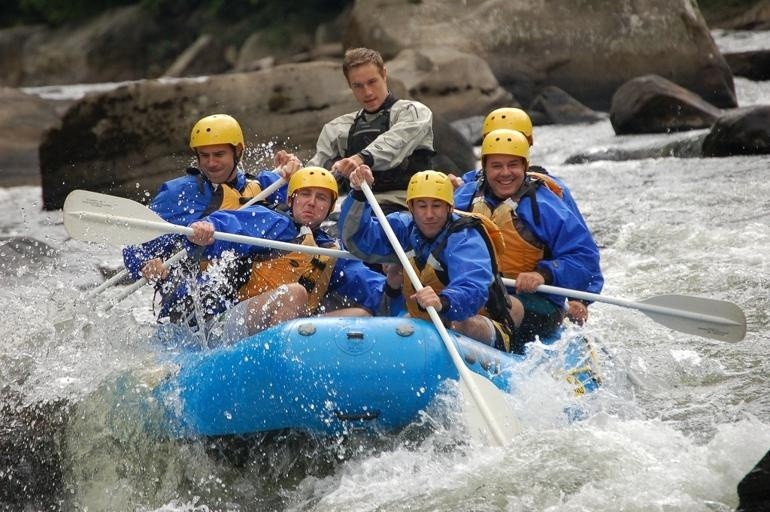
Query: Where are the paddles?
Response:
[349,178,518,448]
[62,189,746,344]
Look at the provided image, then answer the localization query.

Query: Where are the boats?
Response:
[135,314,607,465]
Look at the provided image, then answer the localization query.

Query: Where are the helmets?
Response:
[189,114,244,151]
[482,108,533,170]
[287,166,338,210]
[406,169,454,212]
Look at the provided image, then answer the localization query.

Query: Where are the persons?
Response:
[334,165,524,357]
[303,47,437,237]
[448,105,605,328]
[120,114,301,282]
[158,165,404,349]
[453,128,602,342]
